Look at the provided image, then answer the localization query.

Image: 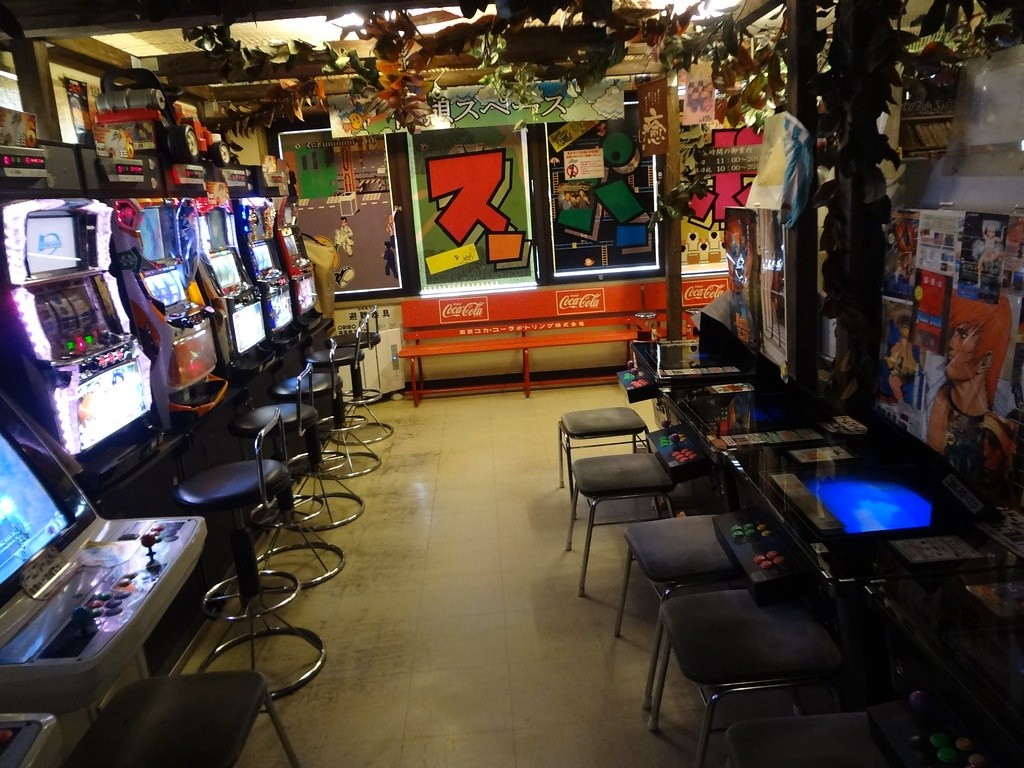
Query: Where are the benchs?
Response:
[398,277,731,407]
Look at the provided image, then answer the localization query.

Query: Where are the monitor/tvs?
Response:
[643,341,730,372]
[787,460,934,537]
[1,395,96,612]
[18,205,293,280]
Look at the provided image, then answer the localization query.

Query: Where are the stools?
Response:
[326,306,395,444]
[304,317,382,480]
[557,408,650,517]
[566,453,675,597]
[174,412,329,695]
[228,362,346,591]
[723,713,913,768]
[62,668,300,768]
[648,590,843,768]
[272,337,365,529]
[613,515,740,707]
